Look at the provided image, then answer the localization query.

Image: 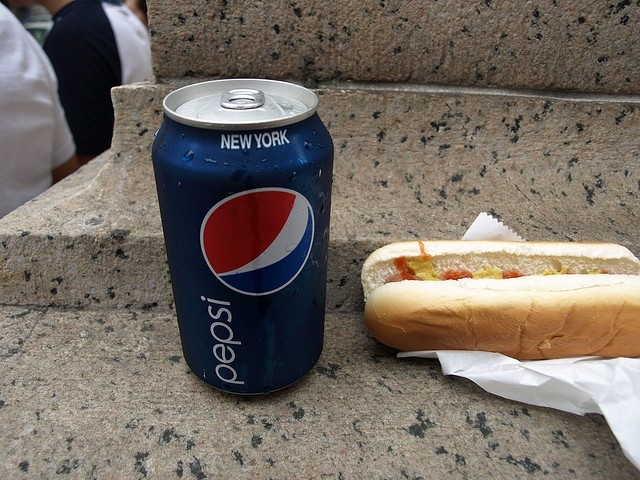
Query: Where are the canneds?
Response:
[151,78,334,397]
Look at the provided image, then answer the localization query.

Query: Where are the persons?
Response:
[11,0,123,166]
[0,1,80,219]
[99,1,154,85]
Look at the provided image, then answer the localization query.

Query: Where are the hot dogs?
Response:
[362,241,640,360]
